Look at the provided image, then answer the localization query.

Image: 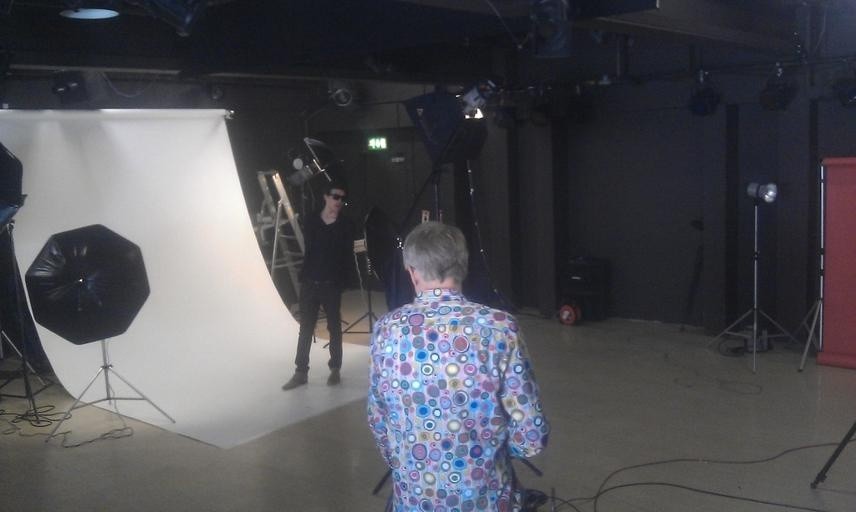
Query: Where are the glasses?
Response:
[327,193,344,202]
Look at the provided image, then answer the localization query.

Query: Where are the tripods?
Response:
[291,187,350,344]
[785,163,824,371]
[45,341,176,445]
[0,226,63,424]
[341,235,381,334]
[707,201,803,375]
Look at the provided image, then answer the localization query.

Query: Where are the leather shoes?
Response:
[282,373,307,390]
[326,371,339,385]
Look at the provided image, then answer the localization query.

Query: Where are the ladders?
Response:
[254,171,306,320]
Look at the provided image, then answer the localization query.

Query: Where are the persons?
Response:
[366,219,550,511]
[281,180,357,392]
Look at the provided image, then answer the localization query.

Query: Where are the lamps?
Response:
[685,60,854,118]
[325,77,353,109]
[701,176,803,371]
